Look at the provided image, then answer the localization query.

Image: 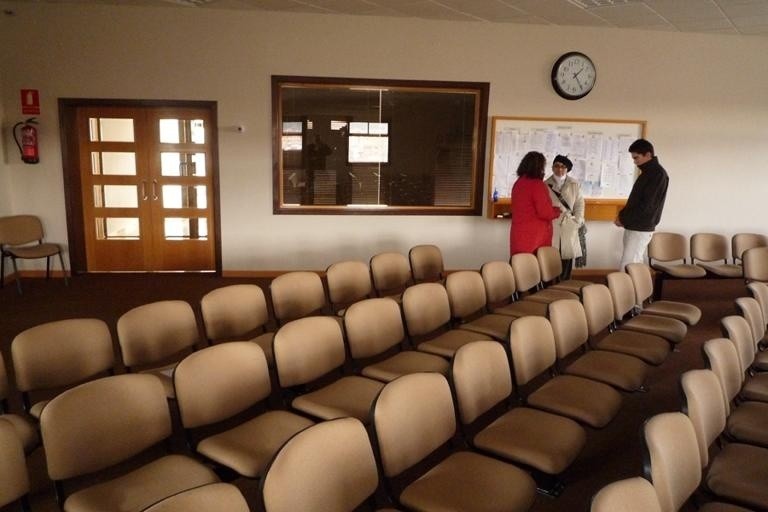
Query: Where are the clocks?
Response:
[551,52,595,101]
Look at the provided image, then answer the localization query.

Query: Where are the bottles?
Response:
[493,186,497,201]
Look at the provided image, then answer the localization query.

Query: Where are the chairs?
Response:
[0,215,69,296]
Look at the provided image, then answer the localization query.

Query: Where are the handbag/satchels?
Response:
[575,225,587,268]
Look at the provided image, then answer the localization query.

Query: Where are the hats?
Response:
[554,154,573,172]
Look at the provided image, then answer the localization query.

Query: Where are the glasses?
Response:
[553,164,567,168]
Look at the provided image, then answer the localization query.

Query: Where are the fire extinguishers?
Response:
[12,117,40,164]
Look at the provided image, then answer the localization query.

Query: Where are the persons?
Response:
[614,138,670,273]
[544,153,585,280]
[509,151,562,265]
[302,133,333,202]
